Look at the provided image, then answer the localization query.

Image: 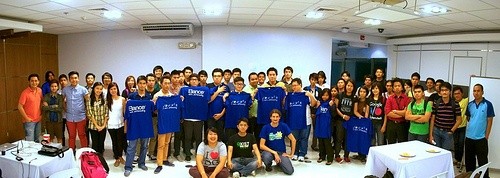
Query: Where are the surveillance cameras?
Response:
[378,29,384,33]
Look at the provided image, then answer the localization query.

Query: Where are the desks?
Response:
[365,140,455,178]
[0,140,76,178]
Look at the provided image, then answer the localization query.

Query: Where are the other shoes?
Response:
[344,158,350,162]
[457,162,461,168]
[298,156,304,161]
[335,157,343,163]
[292,155,297,160]
[154,166,162,174]
[185,156,191,163]
[362,159,366,164]
[326,162,331,165]
[251,170,255,176]
[164,160,173,166]
[174,154,183,161]
[118,156,125,164]
[114,159,120,167]
[311,144,318,151]
[233,171,239,178]
[149,154,157,160]
[317,157,325,163]
[266,162,272,172]
[124,170,130,177]
[139,165,148,170]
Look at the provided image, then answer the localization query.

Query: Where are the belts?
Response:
[436,126,451,132]
[392,121,401,123]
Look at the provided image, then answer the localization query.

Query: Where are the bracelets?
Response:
[450,130,453,134]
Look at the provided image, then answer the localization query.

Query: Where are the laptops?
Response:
[0,143,17,152]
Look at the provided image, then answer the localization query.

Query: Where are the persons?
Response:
[465,84,495,178]
[189,127,229,178]
[404,85,433,143]
[385,79,408,143]
[18,70,494,178]
[227,117,262,178]
[259,108,296,175]
[430,82,462,152]
[452,86,469,173]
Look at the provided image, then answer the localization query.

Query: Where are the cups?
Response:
[43,134,50,144]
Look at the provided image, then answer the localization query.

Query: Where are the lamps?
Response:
[352,0,421,22]
[178,41,196,49]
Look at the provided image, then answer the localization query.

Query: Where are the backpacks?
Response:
[80,151,109,178]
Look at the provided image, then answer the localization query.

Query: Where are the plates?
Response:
[426,149,442,153]
[401,152,416,157]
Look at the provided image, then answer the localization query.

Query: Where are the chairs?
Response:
[470,162,491,178]
[50,147,96,178]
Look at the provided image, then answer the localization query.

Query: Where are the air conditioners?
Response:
[141,24,194,37]
